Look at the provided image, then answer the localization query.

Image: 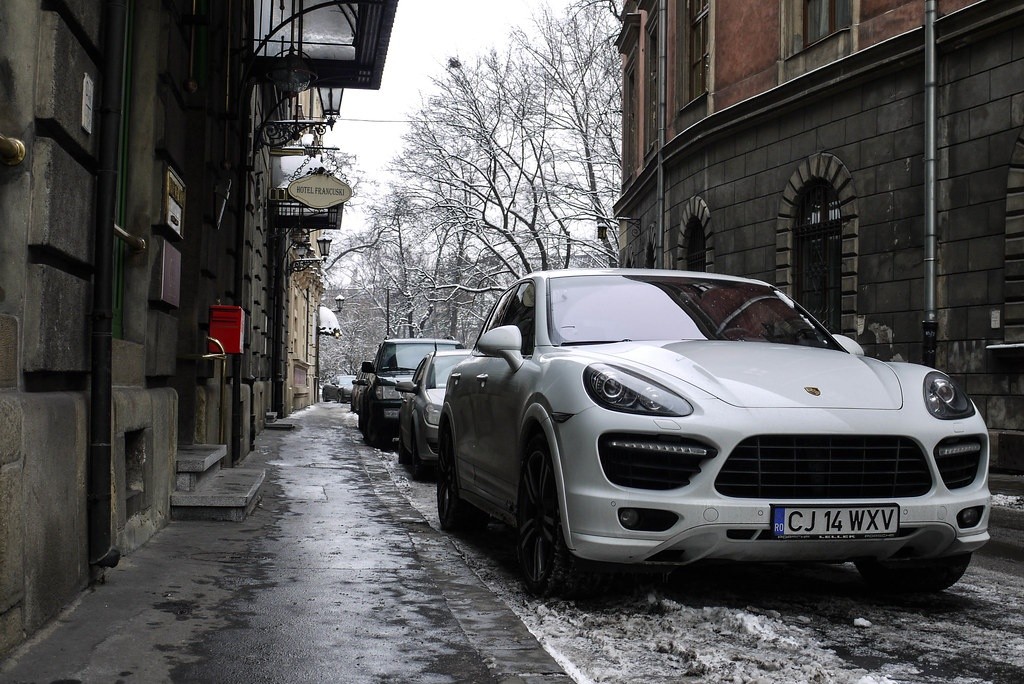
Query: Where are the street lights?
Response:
[386,285,395,335]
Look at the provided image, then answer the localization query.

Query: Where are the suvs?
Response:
[362,336,466,449]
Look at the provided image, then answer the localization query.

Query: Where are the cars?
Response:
[436,269,992,600]
[351,358,375,430]
[322,374,358,403]
[395,349,473,483]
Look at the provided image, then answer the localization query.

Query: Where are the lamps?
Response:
[290,231,332,275]
[253,45,319,164]
[254,87,344,147]
[331,295,344,312]
